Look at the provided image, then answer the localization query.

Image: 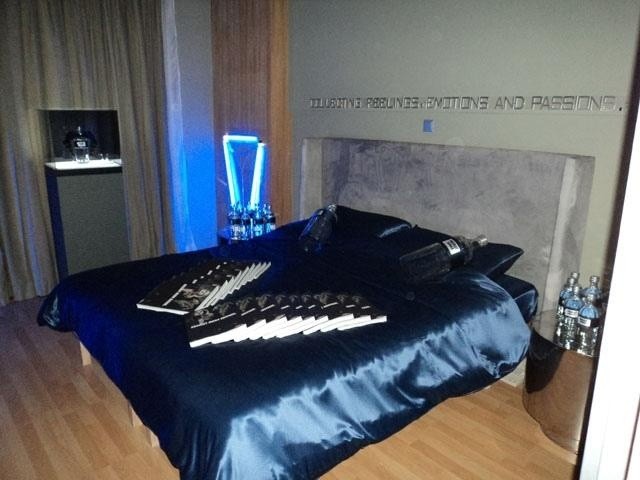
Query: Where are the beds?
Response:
[61,218,538,480]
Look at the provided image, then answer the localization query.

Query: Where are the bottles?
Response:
[400,233,488,281]
[71,127,91,162]
[557,272,600,351]
[225,203,274,239]
[298,202,338,255]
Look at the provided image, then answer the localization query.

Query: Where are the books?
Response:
[133,256,388,349]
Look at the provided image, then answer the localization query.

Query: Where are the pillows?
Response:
[284,203,524,280]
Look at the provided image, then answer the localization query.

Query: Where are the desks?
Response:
[524,307,599,455]
